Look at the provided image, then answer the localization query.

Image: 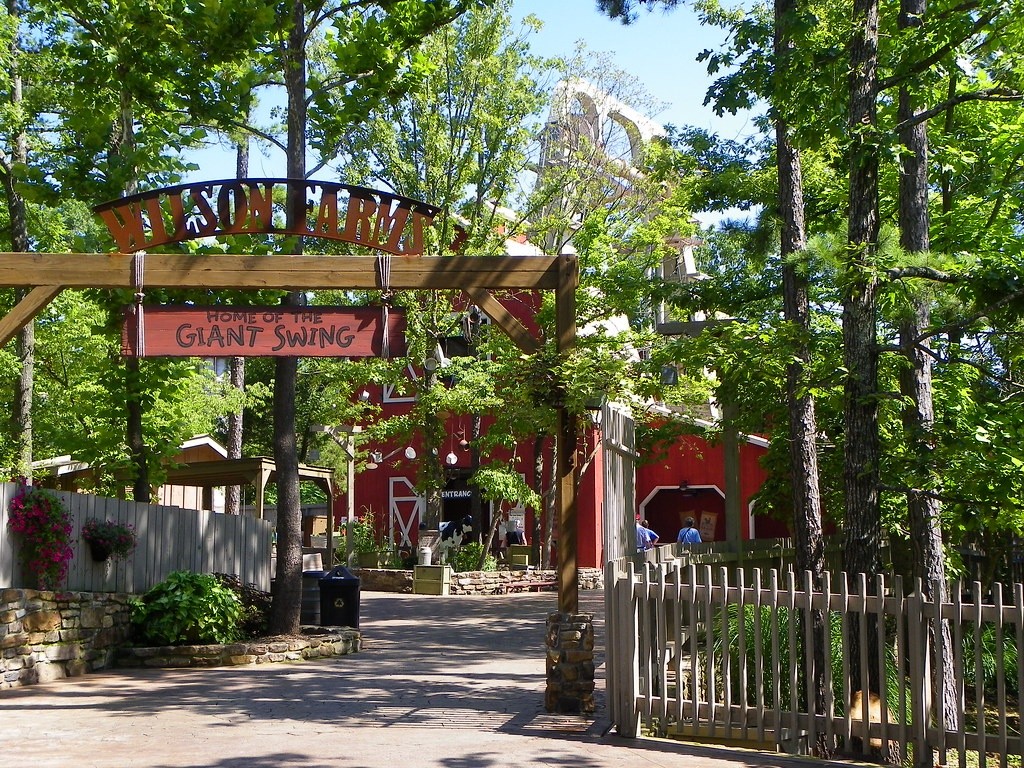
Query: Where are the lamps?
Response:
[680,480,689,491]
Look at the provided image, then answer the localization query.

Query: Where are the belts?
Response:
[637,547,644,548]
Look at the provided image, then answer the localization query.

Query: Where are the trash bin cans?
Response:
[317,565,361,629]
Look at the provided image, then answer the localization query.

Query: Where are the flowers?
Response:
[81,517,138,577]
[357,504,391,553]
[7,475,77,602]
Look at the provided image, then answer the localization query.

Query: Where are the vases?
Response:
[358,553,401,569]
[32,562,51,592]
[90,543,110,563]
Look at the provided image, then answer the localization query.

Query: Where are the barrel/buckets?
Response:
[301,571,325,626]
[418,546,432,566]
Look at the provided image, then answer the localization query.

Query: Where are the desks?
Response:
[509,545,542,570]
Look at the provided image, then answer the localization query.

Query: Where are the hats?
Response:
[636,514,640,520]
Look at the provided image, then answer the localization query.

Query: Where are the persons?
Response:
[636,514,660,554]
[678,516,702,543]
[459,514,473,544]
[502,519,528,546]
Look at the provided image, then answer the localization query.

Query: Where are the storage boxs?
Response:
[512,555,528,565]
[413,564,450,595]
